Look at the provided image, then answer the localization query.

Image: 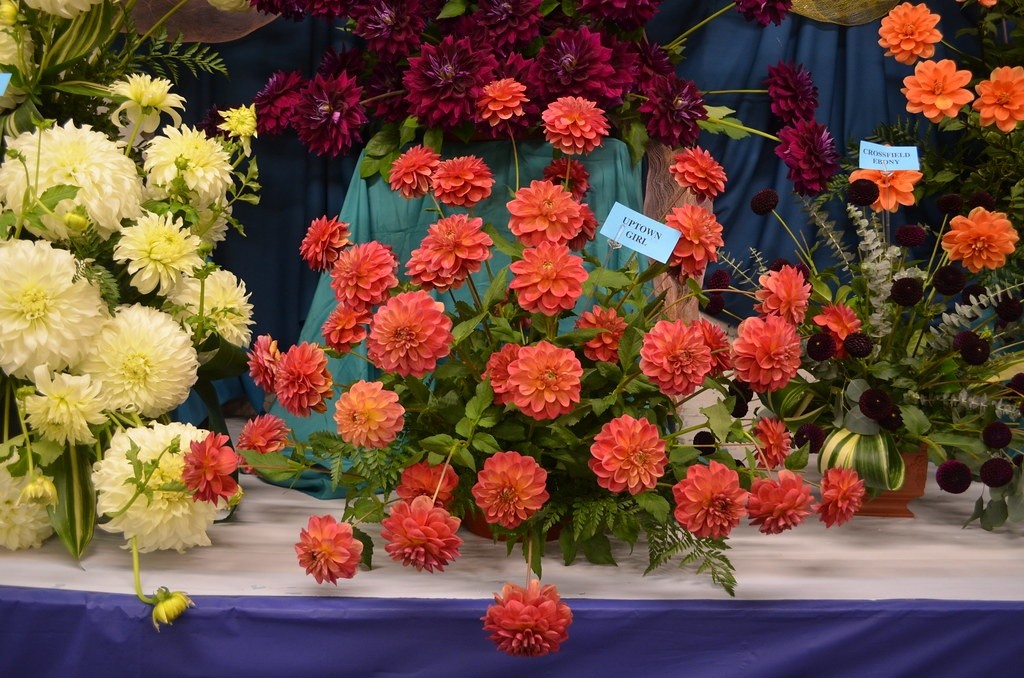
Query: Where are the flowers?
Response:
[2,0,1023,659]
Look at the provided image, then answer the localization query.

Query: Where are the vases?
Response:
[851,441,930,517]
[462,509,573,544]
